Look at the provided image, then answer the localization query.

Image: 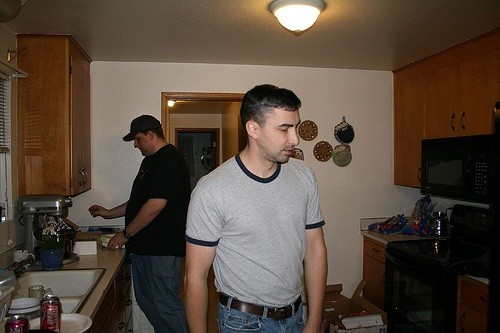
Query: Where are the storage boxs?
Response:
[321,284,387,333]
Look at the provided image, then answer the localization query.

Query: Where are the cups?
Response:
[42,248,64,271]
[14,250,35,263]
[29,284,44,299]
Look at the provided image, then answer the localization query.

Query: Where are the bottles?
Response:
[433,212,448,237]
[7,298,42,333]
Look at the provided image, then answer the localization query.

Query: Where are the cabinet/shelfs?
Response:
[16,28,92,198]
[454,276,488,333]
[361,236,386,310]
[392,27,500,190]
[89,260,134,333]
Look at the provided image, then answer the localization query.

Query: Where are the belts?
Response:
[218,293,301,320]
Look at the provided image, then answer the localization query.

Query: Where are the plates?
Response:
[29,313,93,333]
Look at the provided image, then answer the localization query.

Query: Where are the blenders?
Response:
[20,196,80,264]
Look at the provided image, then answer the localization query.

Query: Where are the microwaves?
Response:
[419,134,500,206]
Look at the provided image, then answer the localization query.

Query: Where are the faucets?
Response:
[7,258,35,277]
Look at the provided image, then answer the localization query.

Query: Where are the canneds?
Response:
[4,314,29,333]
[39,294,62,333]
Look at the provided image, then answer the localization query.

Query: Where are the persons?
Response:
[186,85,328,333]
[88,114,190,333]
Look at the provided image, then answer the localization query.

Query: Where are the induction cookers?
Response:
[384,204,500,286]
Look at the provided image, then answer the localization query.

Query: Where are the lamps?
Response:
[267,0,326,34]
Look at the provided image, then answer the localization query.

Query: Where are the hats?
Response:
[123,115,161,142]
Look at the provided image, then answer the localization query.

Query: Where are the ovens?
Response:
[383,259,457,333]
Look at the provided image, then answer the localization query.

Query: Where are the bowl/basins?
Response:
[37,229,76,258]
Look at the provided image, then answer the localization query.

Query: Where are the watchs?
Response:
[123,229,130,238]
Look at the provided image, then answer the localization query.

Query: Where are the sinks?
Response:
[15,267,106,298]
[58,297,85,314]
